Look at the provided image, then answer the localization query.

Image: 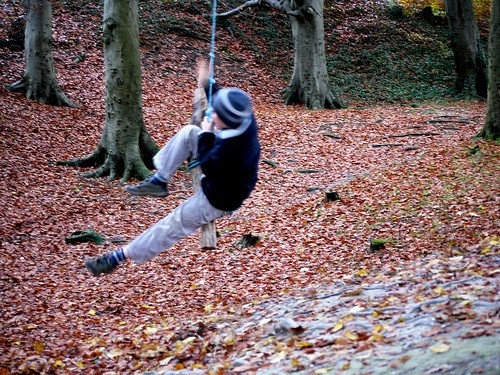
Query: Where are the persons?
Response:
[84,58,260,277]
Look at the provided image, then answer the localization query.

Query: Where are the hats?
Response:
[212,88,252,127]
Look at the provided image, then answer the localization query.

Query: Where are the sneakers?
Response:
[85,255,114,275]
[124,174,169,197]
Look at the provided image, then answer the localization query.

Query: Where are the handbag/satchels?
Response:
[66,229,106,244]
